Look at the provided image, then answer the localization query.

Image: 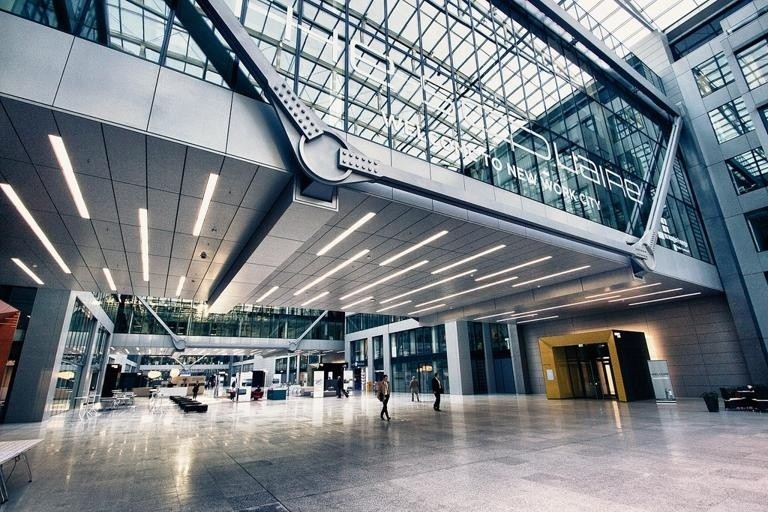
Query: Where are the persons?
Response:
[431,373,442,411]
[410,375,420,402]
[192,381,199,398]
[336,376,343,398]
[375,375,391,421]
[228,381,237,402]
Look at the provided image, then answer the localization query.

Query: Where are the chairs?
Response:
[73,386,170,422]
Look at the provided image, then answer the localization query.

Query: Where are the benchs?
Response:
[170,396,208,414]
[0,439,46,502]
